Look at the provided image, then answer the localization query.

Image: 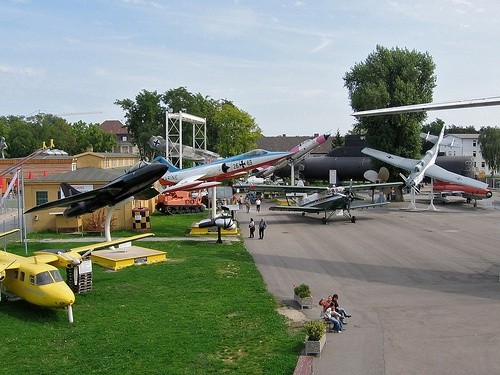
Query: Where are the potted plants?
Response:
[293,283,313,310]
[301,319,327,359]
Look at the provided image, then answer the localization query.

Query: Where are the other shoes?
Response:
[331,323,347,334]
[345,315,351,318]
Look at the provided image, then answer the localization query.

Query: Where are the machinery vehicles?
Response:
[155,191,206,215]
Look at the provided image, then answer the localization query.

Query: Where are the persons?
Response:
[248,218,254,238]
[319,294,332,307]
[330,302,345,330]
[256,198,261,212]
[238,196,243,209]
[330,294,351,324]
[245,197,251,213]
[323,302,342,333]
[258,217,267,240]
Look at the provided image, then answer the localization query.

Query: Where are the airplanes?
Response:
[150,147,299,195]
[360,123,490,194]
[248,132,331,179]
[433,178,493,207]
[0,226,156,308]
[231,181,404,225]
[22,158,169,219]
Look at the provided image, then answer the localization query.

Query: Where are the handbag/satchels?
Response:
[251,227,255,232]
